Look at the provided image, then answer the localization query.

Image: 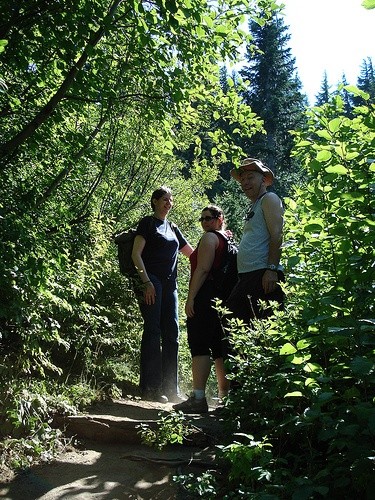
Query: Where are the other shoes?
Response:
[141,394,168,403]
[171,395,186,403]
[172,391,208,413]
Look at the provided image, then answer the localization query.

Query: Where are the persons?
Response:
[132,188,233,403]
[172,205,230,414]
[225,158,287,393]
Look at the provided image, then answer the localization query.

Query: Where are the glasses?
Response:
[199,216,218,222]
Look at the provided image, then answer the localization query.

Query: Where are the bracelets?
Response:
[142,281,154,289]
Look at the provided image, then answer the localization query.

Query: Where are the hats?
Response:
[231,158,273,186]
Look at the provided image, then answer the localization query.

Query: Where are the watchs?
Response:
[267,263,278,271]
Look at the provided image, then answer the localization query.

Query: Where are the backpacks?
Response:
[114,229,138,278]
[207,229,239,299]
